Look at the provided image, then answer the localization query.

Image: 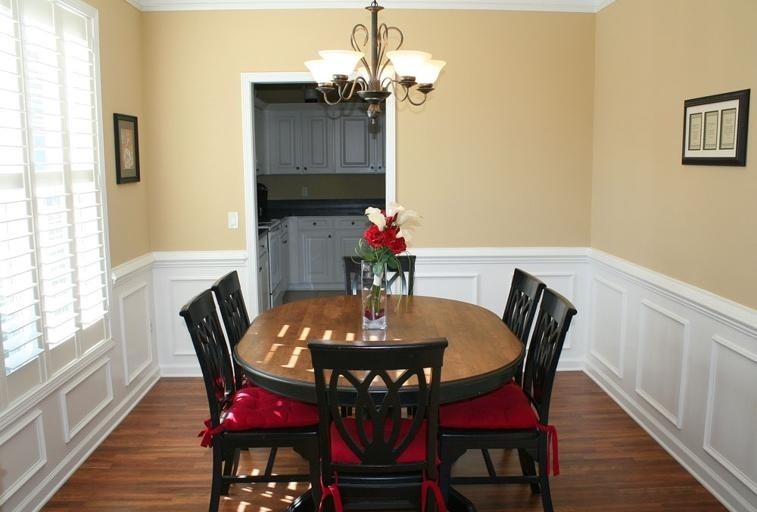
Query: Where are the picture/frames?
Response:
[112,112,140,185]
[681,88,750,167]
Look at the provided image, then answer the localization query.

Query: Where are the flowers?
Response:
[349,204,424,320]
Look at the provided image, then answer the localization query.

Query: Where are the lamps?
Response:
[302,0,447,127]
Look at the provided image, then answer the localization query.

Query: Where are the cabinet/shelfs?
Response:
[257,215,372,314]
[254,96,385,177]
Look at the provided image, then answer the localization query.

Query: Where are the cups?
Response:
[358,259,390,330]
